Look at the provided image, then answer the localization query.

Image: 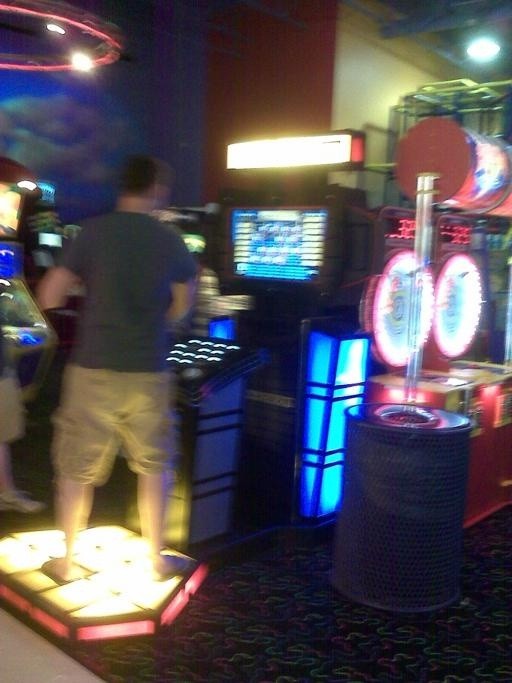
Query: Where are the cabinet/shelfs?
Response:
[365,360,512,528]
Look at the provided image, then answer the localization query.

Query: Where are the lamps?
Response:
[466,27,501,63]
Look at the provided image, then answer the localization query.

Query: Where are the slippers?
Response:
[151,555,187,582]
[41,559,75,586]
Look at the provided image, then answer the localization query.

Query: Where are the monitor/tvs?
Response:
[224,203,345,288]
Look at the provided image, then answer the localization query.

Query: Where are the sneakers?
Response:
[0,490,42,514]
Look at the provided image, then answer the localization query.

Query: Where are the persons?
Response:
[38,151,202,584]
[1,275,59,515]
